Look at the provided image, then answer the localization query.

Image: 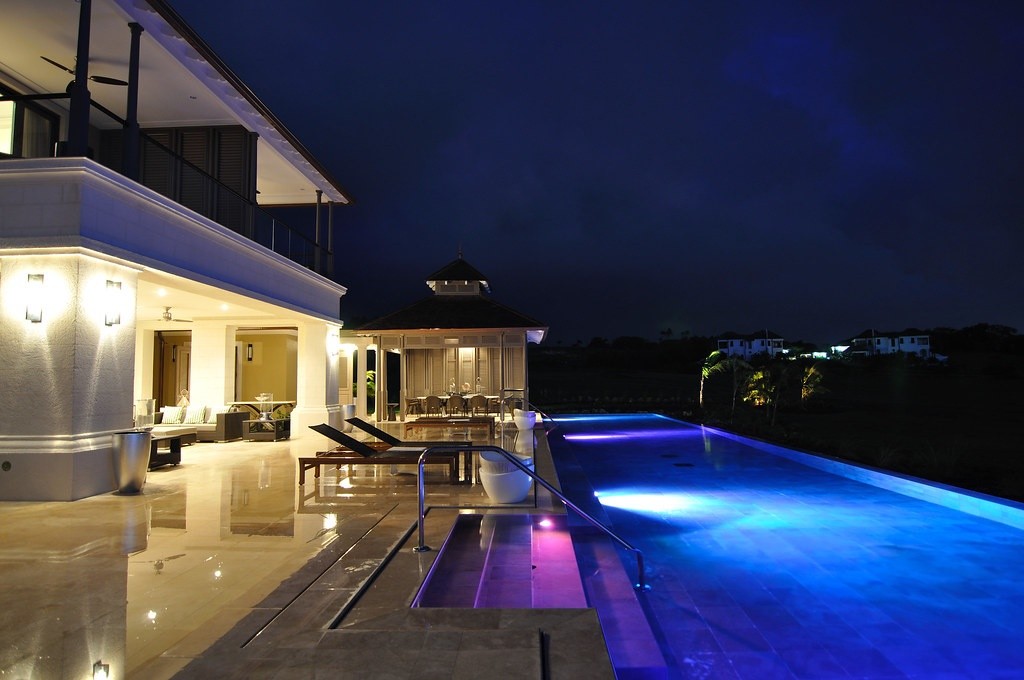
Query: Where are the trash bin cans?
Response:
[112,431,151,494]
[340,404,356,433]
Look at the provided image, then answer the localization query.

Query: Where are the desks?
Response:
[228,400,296,431]
[418,396,500,417]
[243,419,289,441]
[404,417,496,443]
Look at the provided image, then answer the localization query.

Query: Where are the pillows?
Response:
[207,405,231,424]
[161,405,183,424]
[184,404,206,424]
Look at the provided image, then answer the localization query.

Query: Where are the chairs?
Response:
[300,394,515,486]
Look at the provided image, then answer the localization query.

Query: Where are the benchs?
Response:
[148,427,197,471]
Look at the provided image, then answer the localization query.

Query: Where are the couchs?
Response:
[151,405,250,442]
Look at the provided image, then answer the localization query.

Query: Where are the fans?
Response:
[143,307,193,323]
